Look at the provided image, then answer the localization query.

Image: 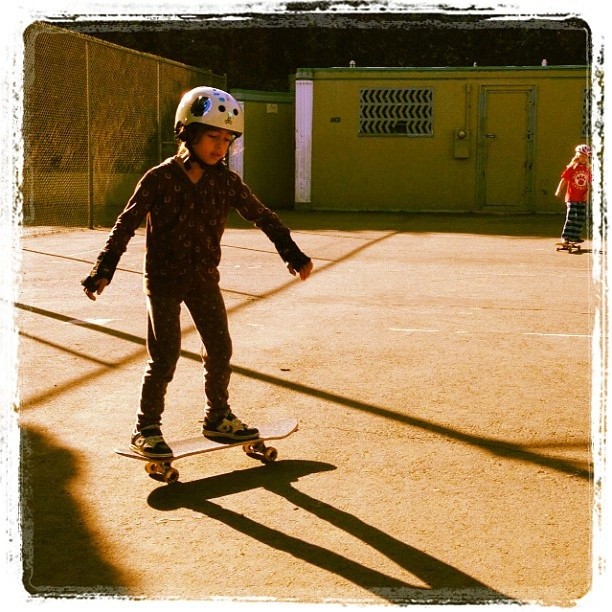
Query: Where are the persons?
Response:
[83,84,315,458]
[552,142,594,243]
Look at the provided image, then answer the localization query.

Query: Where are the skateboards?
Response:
[554,239,585,253]
[114,419,299,485]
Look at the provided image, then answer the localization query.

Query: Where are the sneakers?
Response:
[568,236,584,244]
[201,404,260,440]
[129,421,173,458]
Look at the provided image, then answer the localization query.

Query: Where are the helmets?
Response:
[575,144,591,157]
[173,85,243,138]
